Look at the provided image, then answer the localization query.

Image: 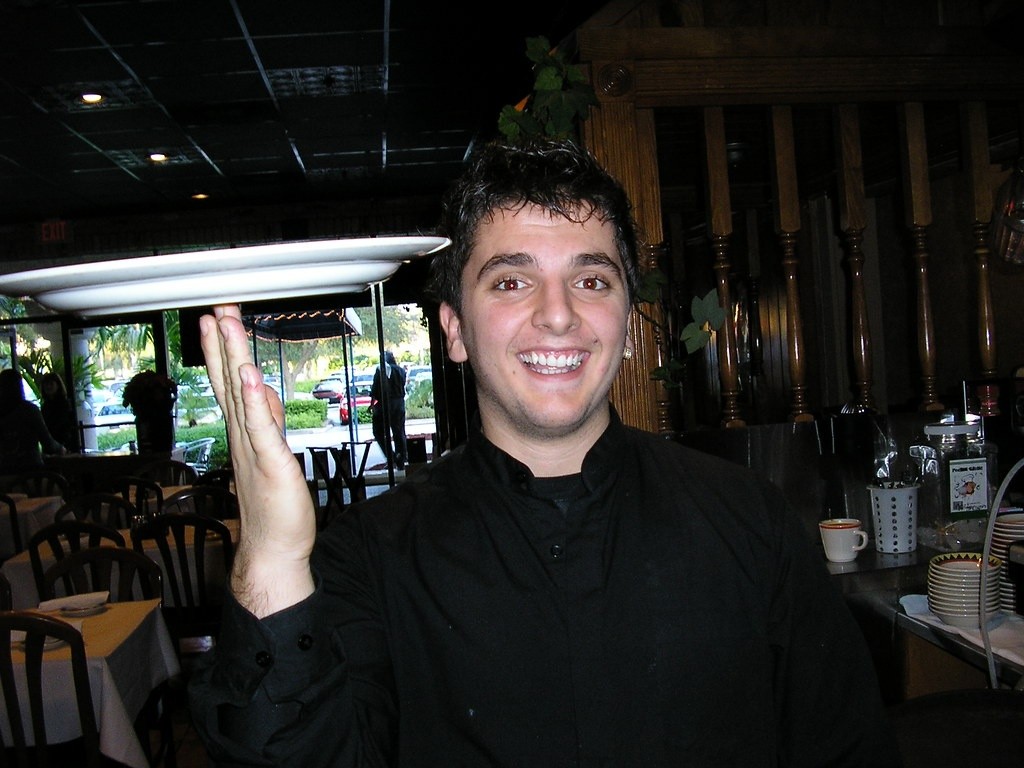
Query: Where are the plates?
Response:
[61,599,107,617]
[20,622,81,652]
[1,237,455,315]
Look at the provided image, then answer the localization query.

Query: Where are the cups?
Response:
[819,519,868,563]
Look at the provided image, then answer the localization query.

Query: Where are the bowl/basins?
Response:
[986,510,1024,611]
[927,552,1001,628]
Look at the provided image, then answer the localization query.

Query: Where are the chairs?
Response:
[0,440,371,768]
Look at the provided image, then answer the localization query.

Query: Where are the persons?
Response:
[38,374,79,450]
[370,350,407,469]
[202,140,839,768]
[0,368,64,479]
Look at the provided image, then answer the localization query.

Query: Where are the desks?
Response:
[101,484,196,524]
[848,587,1023,704]
[1,494,75,554]
[0,597,183,768]
[1,519,240,607]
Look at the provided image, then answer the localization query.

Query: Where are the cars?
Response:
[338,380,376,426]
[92,372,284,427]
[313,362,434,409]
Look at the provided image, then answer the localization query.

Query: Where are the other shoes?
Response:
[397,465,405,470]
[384,454,395,469]
